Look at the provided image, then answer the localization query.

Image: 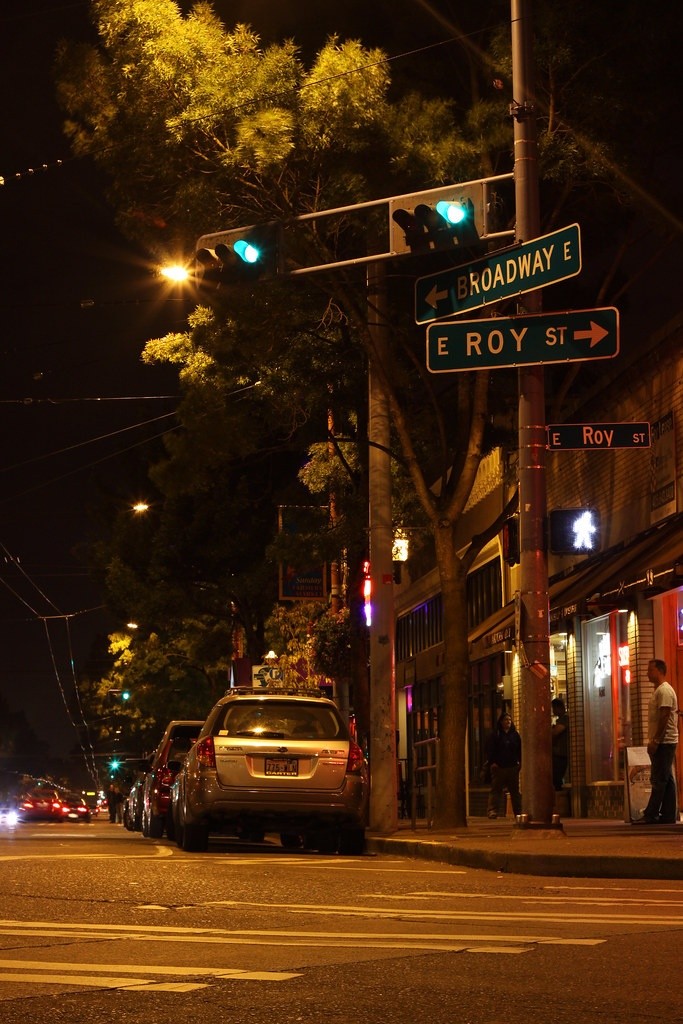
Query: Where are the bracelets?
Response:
[652,739,660,744]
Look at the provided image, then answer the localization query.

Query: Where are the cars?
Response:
[18,787,91,821]
[122,687,371,853]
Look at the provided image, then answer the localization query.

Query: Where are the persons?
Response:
[486,713,522,819]
[108,785,124,824]
[551,698,570,791]
[630,660,679,826]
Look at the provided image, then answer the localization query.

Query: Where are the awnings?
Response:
[467,511,683,667]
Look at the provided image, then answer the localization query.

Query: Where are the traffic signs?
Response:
[425,306,619,374]
[415,222,581,326]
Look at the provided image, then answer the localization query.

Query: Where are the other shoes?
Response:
[631,815,659,825]
[488,810,497,819]
[659,815,676,824]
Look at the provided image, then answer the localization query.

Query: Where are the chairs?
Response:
[239,720,263,734]
[291,725,318,739]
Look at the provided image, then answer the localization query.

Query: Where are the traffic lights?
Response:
[548,507,600,555]
[194,226,271,287]
[390,184,488,252]
